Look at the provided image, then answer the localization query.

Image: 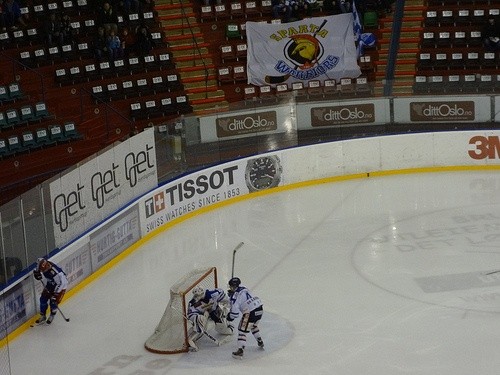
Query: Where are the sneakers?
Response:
[257,337,264,347]
[47,312,56,325]
[232,345,245,359]
[35,314,46,325]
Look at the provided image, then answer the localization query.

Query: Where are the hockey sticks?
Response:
[39,278,70,322]
[230,241,245,312]
[169,304,231,346]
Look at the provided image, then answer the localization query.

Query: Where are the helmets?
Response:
[36,258,50,272]
[192,286,205,300]
[229,277,241,288]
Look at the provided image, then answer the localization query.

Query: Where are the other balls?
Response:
[30,326,34,327]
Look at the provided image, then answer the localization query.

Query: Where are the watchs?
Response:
[243,155,283,194]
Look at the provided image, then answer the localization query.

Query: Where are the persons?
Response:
[185,277,265,360]
[32,258,67,325]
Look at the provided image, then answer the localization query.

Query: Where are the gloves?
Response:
[51,295,56,301]
[34,270,42,280]
[226,311,234,321]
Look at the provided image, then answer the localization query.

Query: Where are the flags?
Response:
[246,13,362,87]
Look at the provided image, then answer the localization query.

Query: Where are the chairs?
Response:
[0,0,193,160]
[413,0,500,95]
[198,0,392,110]
